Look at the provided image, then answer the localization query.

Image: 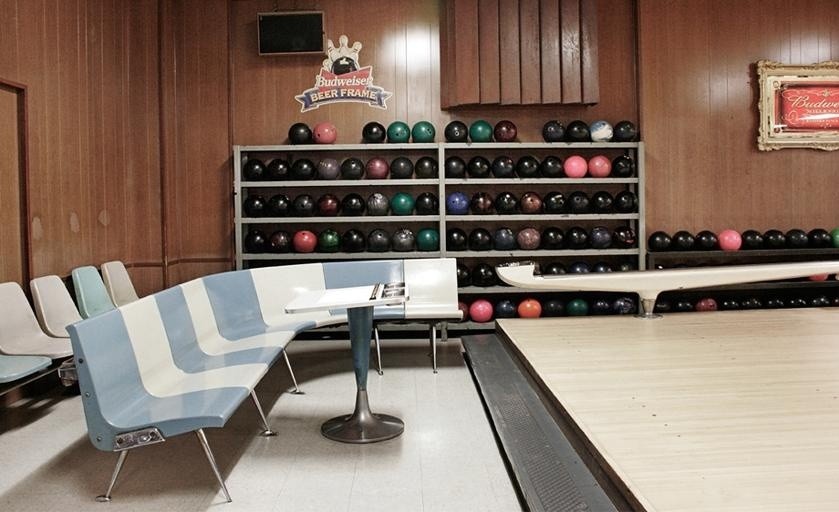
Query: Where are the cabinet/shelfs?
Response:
[645,249,839,308]
[443,143,646,341]
[226,140,442,343]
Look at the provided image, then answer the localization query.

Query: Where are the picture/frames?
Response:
[755,57,839,155]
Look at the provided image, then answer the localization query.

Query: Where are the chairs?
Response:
[0,257,143,440]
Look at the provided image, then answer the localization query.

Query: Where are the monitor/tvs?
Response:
[256,10,327,57]
